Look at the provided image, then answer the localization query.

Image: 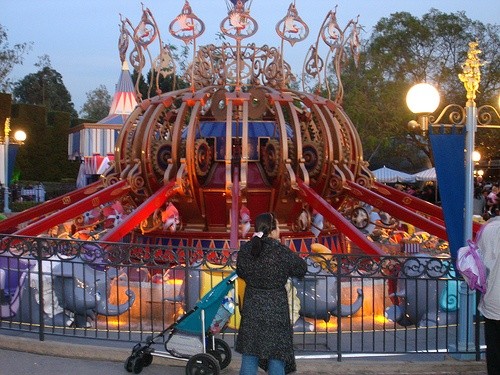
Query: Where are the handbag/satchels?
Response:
[456,218,493,294]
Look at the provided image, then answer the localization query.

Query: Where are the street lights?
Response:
[404,40,500,360]
[3,117,27,214]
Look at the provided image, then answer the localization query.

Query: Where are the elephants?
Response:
[3,254,135,325]
[161,258,364,332]
[385,253,446,328]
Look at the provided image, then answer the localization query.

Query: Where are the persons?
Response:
[474,176,500,217]
[457,216,500,375]
[236,213,308,375]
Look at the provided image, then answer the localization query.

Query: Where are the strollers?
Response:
[124,271,240,375]
